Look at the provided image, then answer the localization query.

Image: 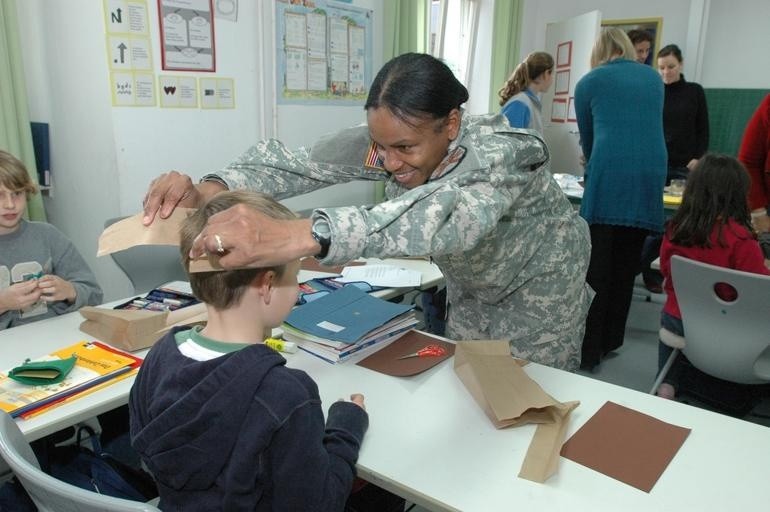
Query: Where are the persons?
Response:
[498,51,554,137]
[0,151,103,331]
[574,28,668,370]
[641,45,709,294]
[128,192,369,512]
[655,154,770,401]
[143,53,596,374]
[627,30,652,63]
[739,94,770,259]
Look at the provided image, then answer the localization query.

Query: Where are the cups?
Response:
[669,178,687,198]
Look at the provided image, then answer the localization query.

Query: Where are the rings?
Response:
[215,235,224,252]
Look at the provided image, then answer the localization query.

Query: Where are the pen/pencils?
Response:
[141,294,183,306]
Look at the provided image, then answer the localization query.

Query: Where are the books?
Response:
[0,340,145,421]
[280,283,421,365]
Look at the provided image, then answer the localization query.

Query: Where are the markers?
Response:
[264,337,297,354]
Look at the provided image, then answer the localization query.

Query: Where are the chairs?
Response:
[106,215,190,294]
[648,254,770,395]
[0,410,160,512]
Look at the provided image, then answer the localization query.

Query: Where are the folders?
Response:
[30,122,49,187]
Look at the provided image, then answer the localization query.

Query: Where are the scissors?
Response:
[397,344,446,361]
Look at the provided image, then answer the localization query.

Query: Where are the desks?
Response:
[277,331,770,512]
[563,178,770,258]
[0,253,444,477]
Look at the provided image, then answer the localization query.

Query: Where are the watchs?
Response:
[311,217,331,260]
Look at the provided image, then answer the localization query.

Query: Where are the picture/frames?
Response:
[601,17,663,69]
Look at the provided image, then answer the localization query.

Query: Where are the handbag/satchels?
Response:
[50,426,159,502]
